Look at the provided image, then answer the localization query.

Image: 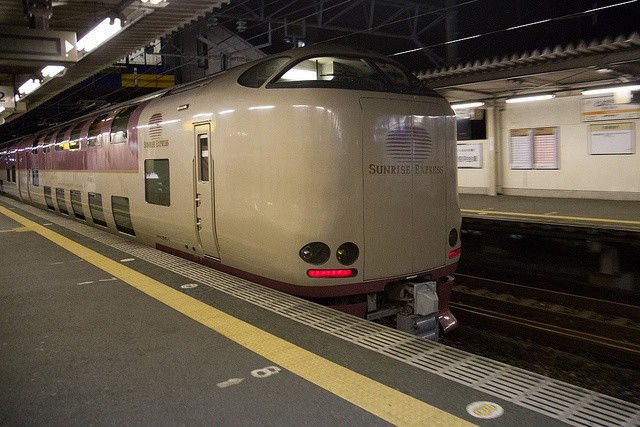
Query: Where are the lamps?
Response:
[17,75,44,97]
[76,10,129,54]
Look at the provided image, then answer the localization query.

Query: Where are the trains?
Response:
[1,44,462,342]
